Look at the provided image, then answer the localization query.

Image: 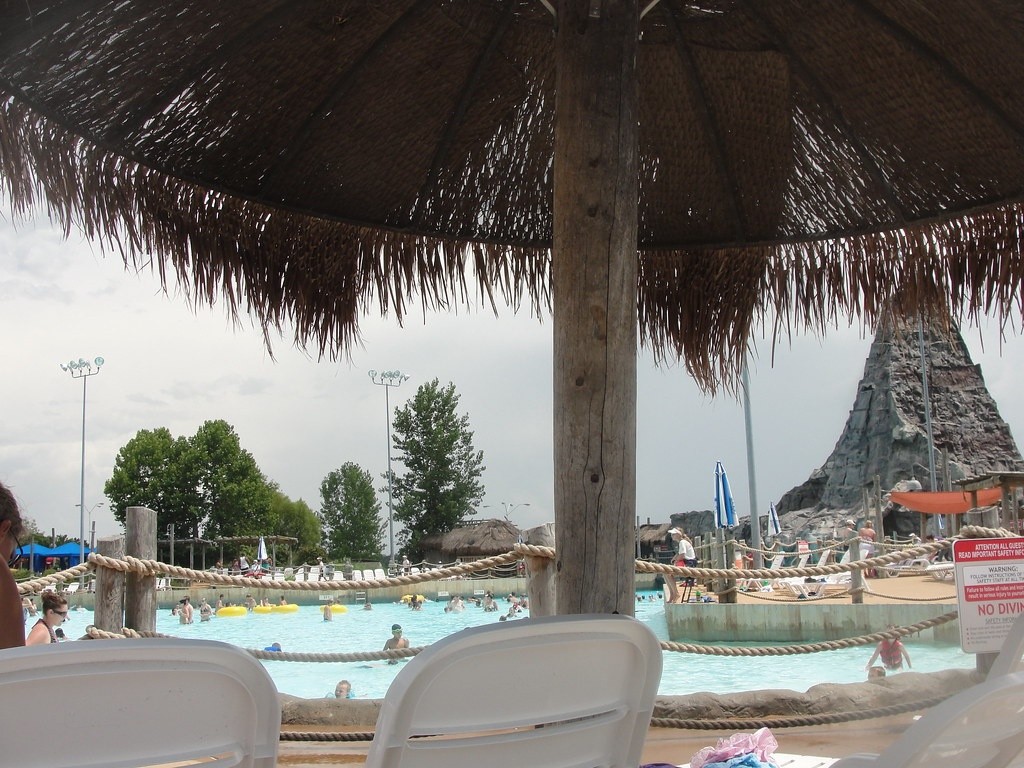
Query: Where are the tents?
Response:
[11,542,96,573]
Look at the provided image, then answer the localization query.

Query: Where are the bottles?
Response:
[696,590,701,601]
[764,579,768,587]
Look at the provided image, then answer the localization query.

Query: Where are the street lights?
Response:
[75,502,104,549]
[483,502,530,519]
[59,356,104,591]
[368,368,410,574]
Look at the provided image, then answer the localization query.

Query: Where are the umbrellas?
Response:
[713,462,740,531]
[0,1,1024,631]
[768,502,783,536]
[257,536,268,560]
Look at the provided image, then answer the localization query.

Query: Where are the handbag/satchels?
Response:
[675,560,686,567]
[253,571,259,576]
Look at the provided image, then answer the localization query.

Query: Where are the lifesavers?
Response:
[402,594,424,602]
[320,605,346,613]
[217,607,247,617]
[253,603,298,613]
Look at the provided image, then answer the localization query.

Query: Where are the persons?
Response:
[665,528,698,603]
[865,625,913,682]
[0,484,35,650]
[926,536,951,562]
[171,553,531,665]
[22,590,72,647]
[842,520,876,577]
[334,681,352,701]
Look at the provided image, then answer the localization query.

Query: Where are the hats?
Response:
[317,556,322,559]
[485,594,492,598]
[403,556,407,558]
[909,533,915,536]
[668,528,682,535]
[846,520,856,525]
[253,560,257,563]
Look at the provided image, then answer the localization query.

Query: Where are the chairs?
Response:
[42,567,438,595]
[740,544,955,596]
[365,614,663,768]
[0,638,280,768]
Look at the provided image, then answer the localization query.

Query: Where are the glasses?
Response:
[52,609,68,617]
[392,628,403,632]
[8,527,23,566]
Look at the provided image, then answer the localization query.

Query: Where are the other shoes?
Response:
[679,582,689,587]
